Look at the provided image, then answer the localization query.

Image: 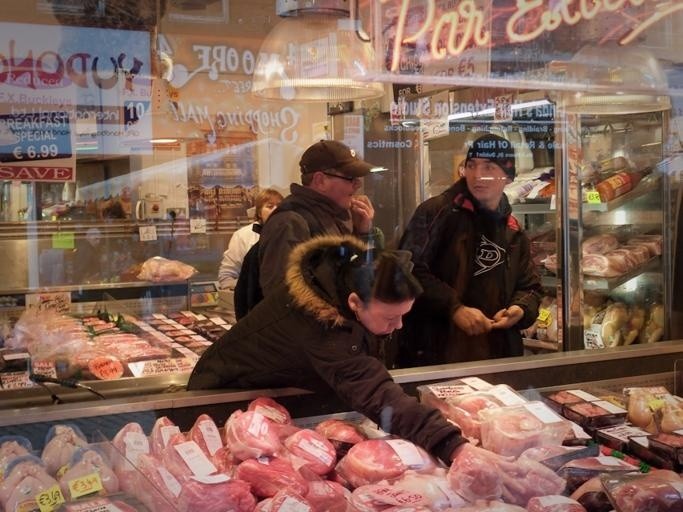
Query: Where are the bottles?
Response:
[580,166,654,204]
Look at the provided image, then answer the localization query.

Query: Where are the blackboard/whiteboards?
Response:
[0,111,72,162]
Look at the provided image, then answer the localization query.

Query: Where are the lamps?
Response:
[251,0,387,104]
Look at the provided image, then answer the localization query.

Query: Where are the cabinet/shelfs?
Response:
[505,84,679,354]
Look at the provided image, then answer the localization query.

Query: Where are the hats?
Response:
[464,133,515,181]
[299,140,374,177]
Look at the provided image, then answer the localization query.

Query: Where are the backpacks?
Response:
[234,204,321,321]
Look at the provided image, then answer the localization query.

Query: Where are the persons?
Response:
[217,187,285,289]
[255,138,377,291]
[397,133,543,364]
[187,235,526,503]
